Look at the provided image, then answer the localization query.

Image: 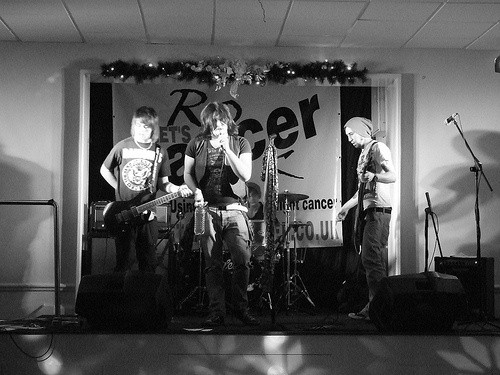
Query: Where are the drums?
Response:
[247,220,267,248]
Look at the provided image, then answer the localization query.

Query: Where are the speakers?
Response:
[434,256,495,320]
[88,233,173,276]
[74,268,175,335]
[367,272,465,333]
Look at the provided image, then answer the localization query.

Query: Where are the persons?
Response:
[334,117,396,320]
[100,106,192,280]
[245,180,280,250]
[184,101,261,328]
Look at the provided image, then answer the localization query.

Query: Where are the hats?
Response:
[245,181,261,199]
[344,117,373,138]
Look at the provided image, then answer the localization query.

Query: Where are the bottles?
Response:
[194,201,205,235]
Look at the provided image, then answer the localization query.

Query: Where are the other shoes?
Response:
[232,311,260,330]
[348,300,370,320]
[200,311,225,329]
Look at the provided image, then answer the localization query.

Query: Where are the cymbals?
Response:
[274,193,310,202]
[281,222,310,228]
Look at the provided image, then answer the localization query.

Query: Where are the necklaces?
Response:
[133,136,152,150]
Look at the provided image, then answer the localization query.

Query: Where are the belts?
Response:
[365,207,391,214]
[209,203,249,213]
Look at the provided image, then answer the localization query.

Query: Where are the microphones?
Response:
[217,130,224,151]
[444,112,458,125]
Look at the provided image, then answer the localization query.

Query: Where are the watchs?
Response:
[371,173,378,183]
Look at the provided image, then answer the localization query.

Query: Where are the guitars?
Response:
[349,151,372,267]
[100,187,181,240]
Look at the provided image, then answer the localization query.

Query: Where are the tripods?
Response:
[273,196,322,316]
